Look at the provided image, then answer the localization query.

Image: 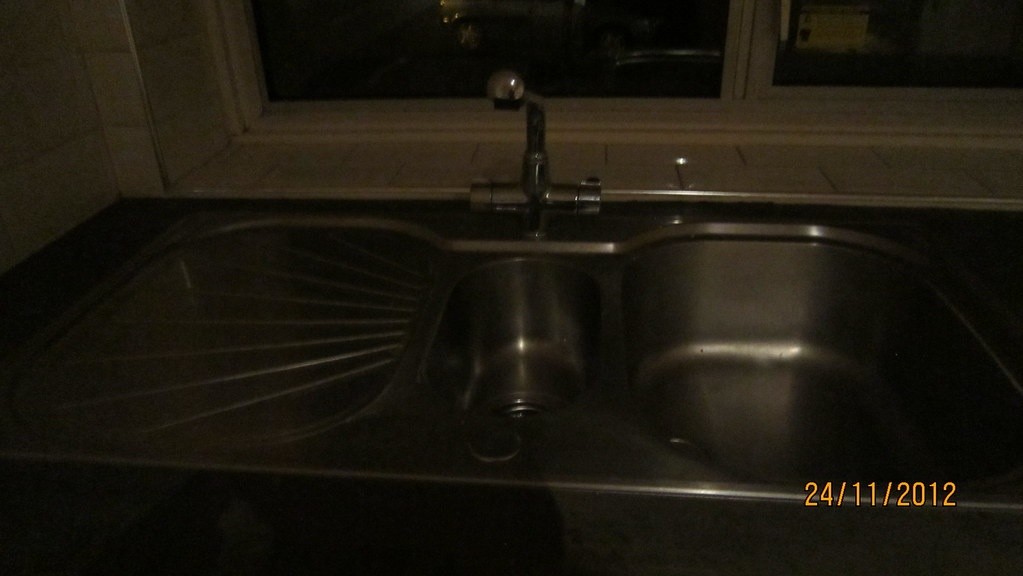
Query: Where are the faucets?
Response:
[482,68,552,243]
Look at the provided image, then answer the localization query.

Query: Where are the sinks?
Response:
[620,213,1023,510]
[0,211,454,467]
[420,252,609,452]
